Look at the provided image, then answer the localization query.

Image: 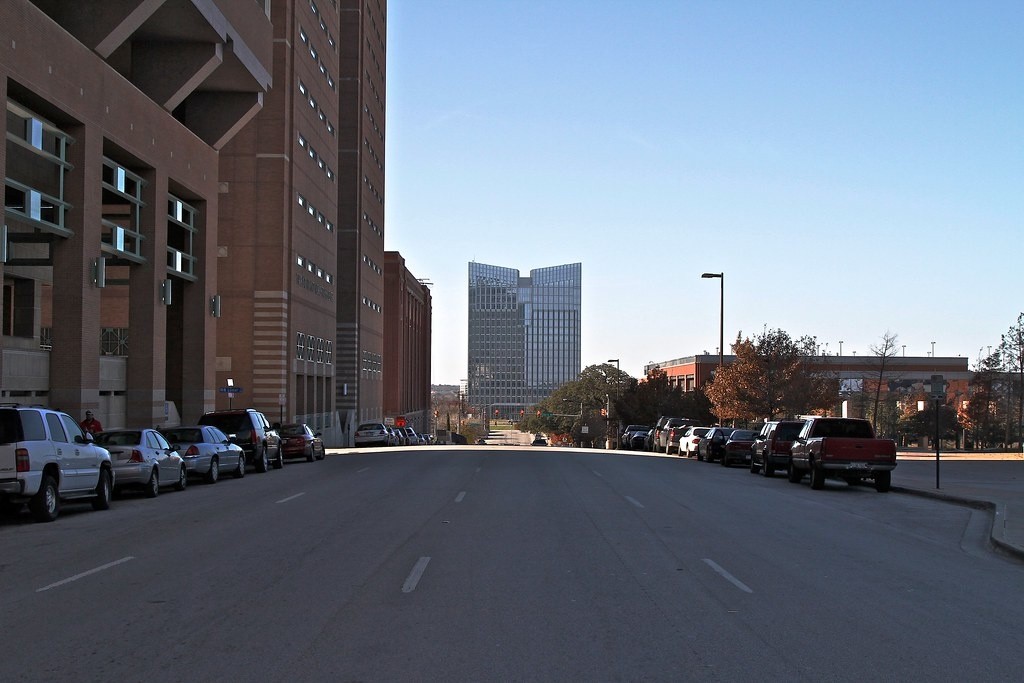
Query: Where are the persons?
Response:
[79,410,103,433]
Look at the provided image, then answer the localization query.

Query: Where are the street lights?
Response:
[931,393,943,489]
[606,359,621,451]
[838,340,843,357]
[901,345,906,356]
[931,341,936,355]
[987,345,992,356]
[927,352,931,357]
[701,272,725,428]
[852,351,857,355]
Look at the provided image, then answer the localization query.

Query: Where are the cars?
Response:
[695,428,736,464]
[676,427,711,459]
[155,425,247,485]
[621,415,703,455]
[719,430,763,467]
[93,428,188,498]
[353,423,435,446]
[277,423,325,464]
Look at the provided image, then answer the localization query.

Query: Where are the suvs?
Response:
[197,409,286,473]
[748,419,806,478]
[0,402,116,521]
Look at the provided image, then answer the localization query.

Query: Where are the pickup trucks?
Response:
[788,417,898,492]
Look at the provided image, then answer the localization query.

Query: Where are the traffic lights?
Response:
[538,410,541,418]
[521,410,524,417]
[495,410,498,416]
[601,409,606,415]
